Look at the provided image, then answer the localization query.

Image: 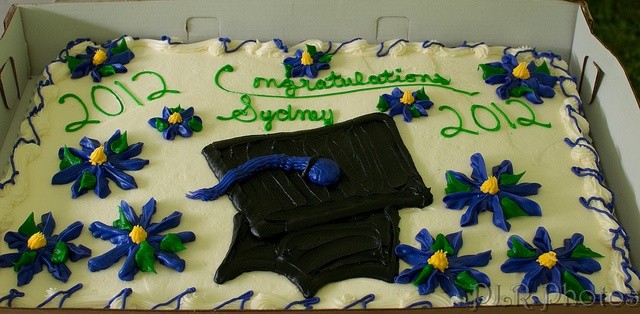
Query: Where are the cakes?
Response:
[1,36,639,311]
